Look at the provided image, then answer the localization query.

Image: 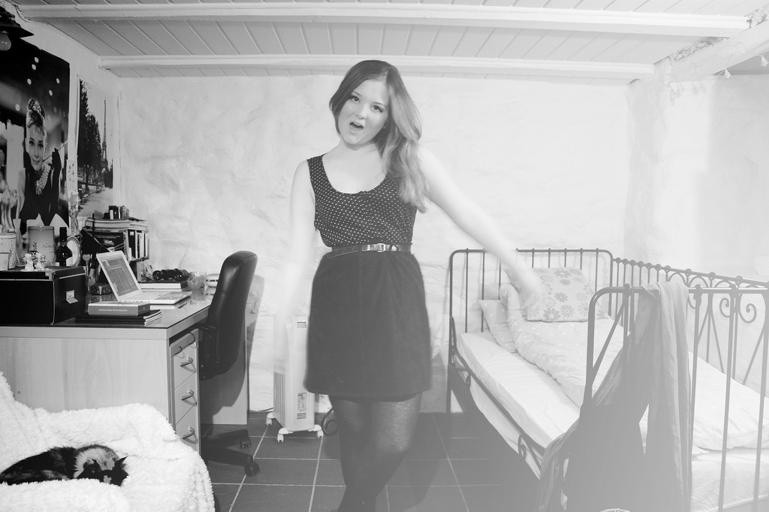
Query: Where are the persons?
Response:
[280,60,548,512]
[6,97,61,235]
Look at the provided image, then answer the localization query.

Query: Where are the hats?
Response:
[26,98,46,135]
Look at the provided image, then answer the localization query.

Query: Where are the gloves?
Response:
[51,148,61,169]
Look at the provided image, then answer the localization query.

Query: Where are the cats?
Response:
[0,443,128,487]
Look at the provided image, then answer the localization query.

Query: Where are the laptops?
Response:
[96,250,193,305]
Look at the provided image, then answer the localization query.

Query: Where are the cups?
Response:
[189,272,208,304]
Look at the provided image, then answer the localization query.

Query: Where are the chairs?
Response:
[200,251,258,477]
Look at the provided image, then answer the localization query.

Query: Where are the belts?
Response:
[327,242,412,259]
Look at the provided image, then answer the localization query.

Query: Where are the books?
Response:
[75,309,162,323]
[139,280,188,289]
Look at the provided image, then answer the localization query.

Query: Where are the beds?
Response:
[449,249,768,505]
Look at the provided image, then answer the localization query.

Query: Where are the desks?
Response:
[0,293,256,451]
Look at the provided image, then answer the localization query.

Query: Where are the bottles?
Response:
[108,205,129,220]
[54,227,73,266]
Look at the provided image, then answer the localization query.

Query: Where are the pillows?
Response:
[478,267,605,353]
